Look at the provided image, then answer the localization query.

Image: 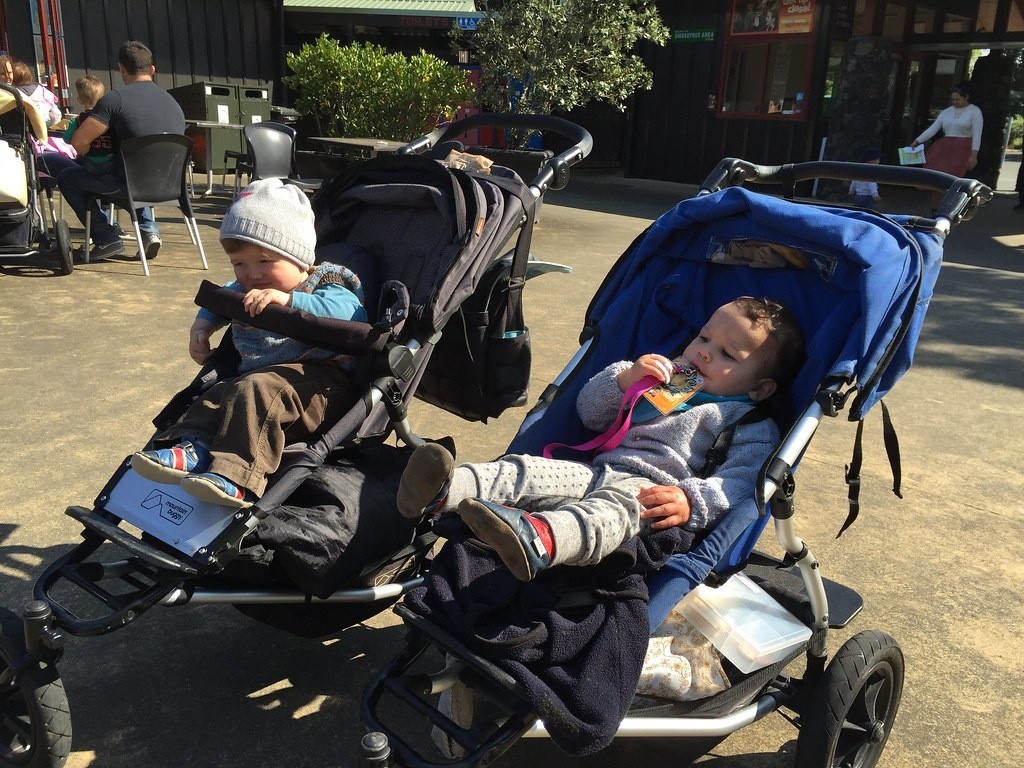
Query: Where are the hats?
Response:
[219,177,318,271]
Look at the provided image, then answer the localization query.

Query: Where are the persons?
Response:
[126,174,372,511]
[0,55,14,87]
[57,40,185,263]
[11,61,63,128]
[396,294,804,594]
[63,75,116,176]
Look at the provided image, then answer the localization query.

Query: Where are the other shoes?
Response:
[456,496,555,583]
[131,440,256,508]
[397,443,454,519]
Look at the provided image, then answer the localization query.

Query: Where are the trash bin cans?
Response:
[166,81,240,175]
[237,84,272,175]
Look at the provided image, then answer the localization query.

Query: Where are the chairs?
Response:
[83,132,208,277]
[243,118,326,193]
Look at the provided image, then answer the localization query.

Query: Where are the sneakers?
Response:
[80,240,125,260]
[135,235,161,260]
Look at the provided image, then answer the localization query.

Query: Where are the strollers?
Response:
[0,112,600,768]
[0,83,74,274]
[358,153,993,767]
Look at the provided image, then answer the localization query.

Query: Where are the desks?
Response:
[307,134,409,163]
